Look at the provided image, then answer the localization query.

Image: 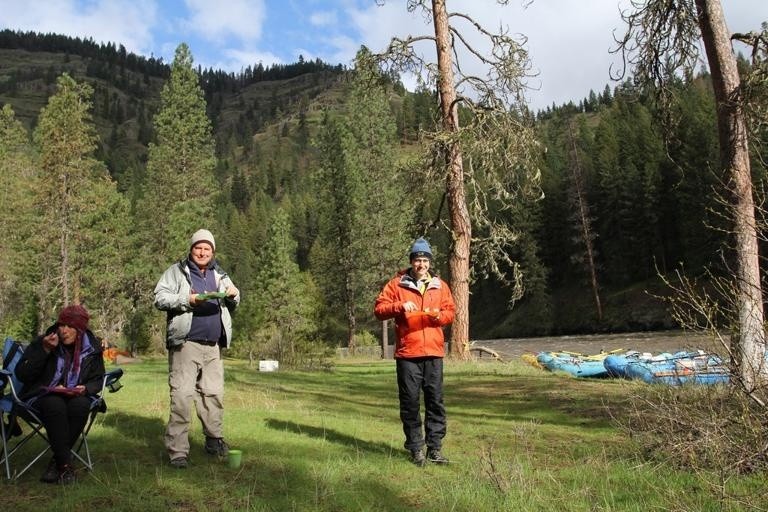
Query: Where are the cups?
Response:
[228,449,243,472]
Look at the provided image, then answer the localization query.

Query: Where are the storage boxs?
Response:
[259,360,278,372]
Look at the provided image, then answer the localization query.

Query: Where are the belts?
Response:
[191,339,216,346]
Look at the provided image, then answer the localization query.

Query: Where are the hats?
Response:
[190,228,216,253]
[56,305,89,334]
[409,237,433,261]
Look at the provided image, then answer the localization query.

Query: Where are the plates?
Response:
[196,292,226,300]
[40,386,84,398]
[406,310,440,317]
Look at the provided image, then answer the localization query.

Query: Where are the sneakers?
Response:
[204,437,229,457]
[39,458,58,482]
[57,467,73,485]
[169,456,187,469]
[426,449,449,464]
[410,451,427,467]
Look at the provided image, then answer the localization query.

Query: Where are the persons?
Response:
[152,227,242,469]
[373,235,459,469]
[15,303,107,485]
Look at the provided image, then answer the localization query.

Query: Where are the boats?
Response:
[520,347,594,371]
[622,348,768,387]
[536,349,638,379]
[601,349,718,379]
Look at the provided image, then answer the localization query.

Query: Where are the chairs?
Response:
[0,335,125,488]
[0,378,15,480]
